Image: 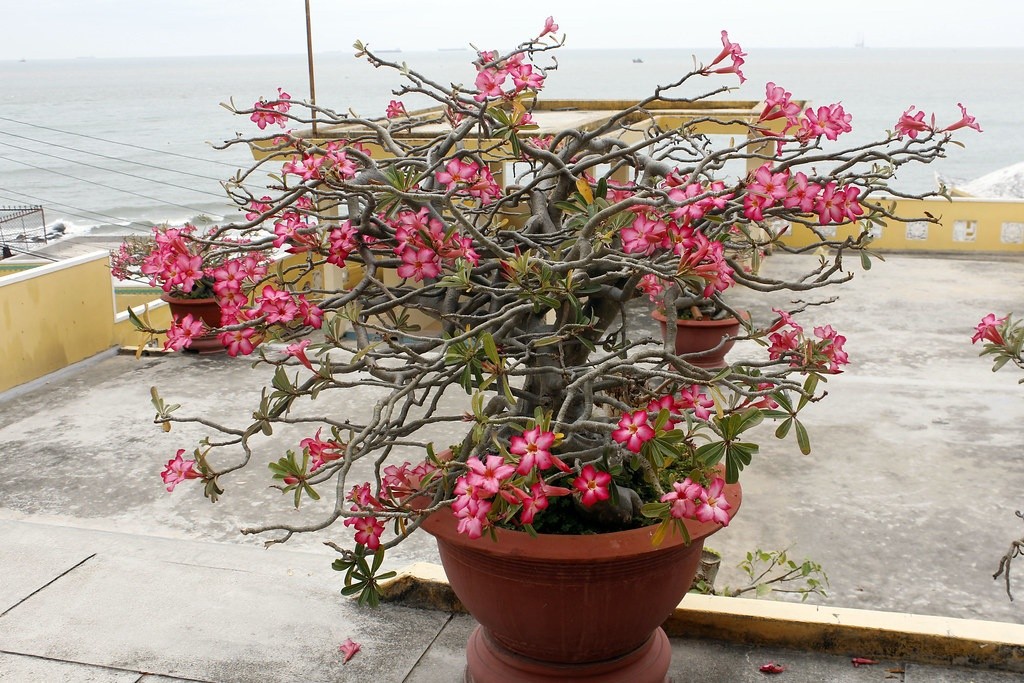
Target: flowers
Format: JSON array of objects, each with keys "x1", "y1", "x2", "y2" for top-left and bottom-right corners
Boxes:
[{"x1": 104, "y1": 11, "x2": 1024, "y2": 608}]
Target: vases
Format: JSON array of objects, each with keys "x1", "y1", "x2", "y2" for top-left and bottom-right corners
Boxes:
[{"x1": 399, "y1": 449, "x2": 742, "y2": 683}]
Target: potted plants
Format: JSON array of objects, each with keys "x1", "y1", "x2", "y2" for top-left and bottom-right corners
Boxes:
[
  {"x1": 650, "y1": 287, "x2": 749, "y2": 373},
  {"x1": 159, "y1": 276, "x2": 227, "y2": 356}
]
